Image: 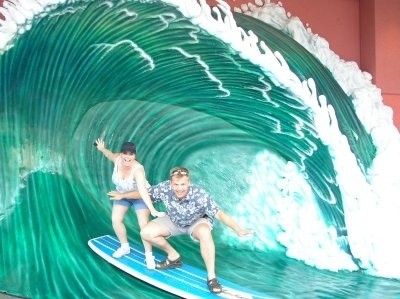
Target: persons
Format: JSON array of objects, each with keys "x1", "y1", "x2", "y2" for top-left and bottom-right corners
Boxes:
[
  {"x1": 96, "y1": 138, "x2": 166, "y2": 270},
  {"x1": 106, "y1": 168, "x2": 256, "y2": 293}
]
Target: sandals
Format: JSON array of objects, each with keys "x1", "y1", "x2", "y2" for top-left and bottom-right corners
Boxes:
[
  {"x1": 155, "y1": 256, "x2": 182, "y2": 269},
  {"x1": 207, "y1": 278, "x2": 222, "y2": 293}
]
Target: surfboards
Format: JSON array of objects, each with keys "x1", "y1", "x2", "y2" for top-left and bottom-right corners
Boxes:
[{"x1": 88, "y1": 234, "x2": 275, "y2": 299}]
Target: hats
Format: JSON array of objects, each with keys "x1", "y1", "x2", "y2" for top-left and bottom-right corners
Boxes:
[{"x1": 121, "y1": 142, "x2": 136, "y2": 153}]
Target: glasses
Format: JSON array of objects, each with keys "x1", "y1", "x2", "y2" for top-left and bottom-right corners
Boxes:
[{"x1": 171, "y1": 172, "x2": 188, "y2": 176}]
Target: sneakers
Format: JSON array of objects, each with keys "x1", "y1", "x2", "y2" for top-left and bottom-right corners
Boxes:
[
  {"x1": 146, "y1": 256, "x2": 155, "y2": 269},
  {"x1": 113, "y1": 246, "x2": 130, "y2": 258}
]
